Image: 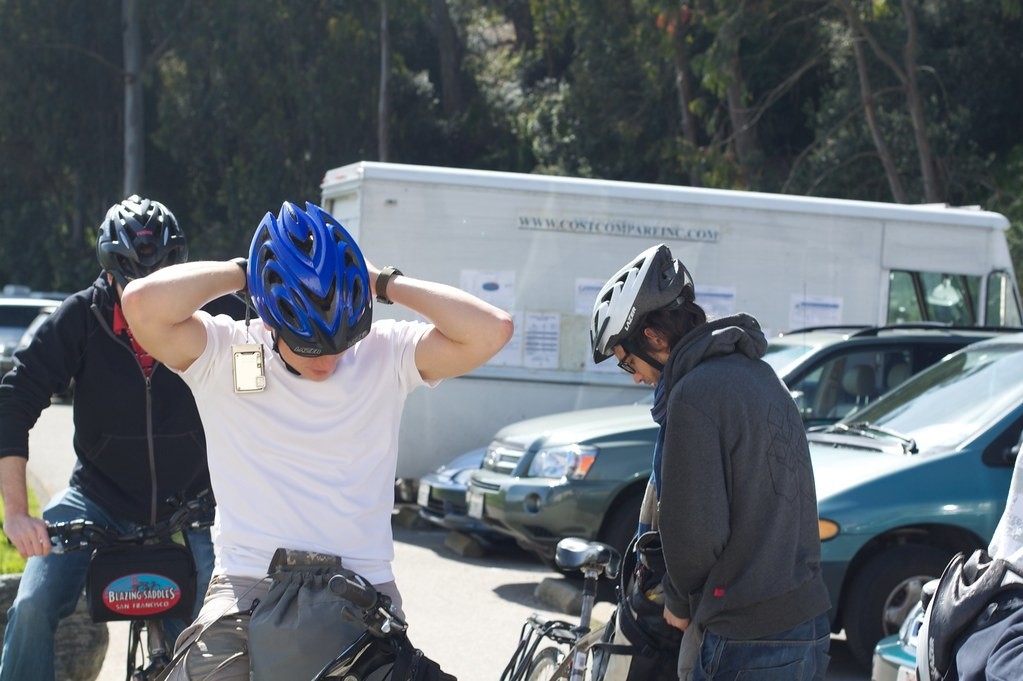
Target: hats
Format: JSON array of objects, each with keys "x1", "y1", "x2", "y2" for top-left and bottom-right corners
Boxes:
[{"x1": 588, "y1": 243, "x2": 695, "y2": 365}]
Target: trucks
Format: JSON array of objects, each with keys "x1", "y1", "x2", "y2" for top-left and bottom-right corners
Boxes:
[{"x1": 316, "y1": 158, "x2": 1022, "y2": 509}]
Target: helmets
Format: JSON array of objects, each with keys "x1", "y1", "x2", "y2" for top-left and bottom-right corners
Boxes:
[
  {"x1": 96, "y1": 193, "x2": 189, "y2": 291},
  {"x1": 245, "y1": 201, "x2": 373, "y2": 358}
]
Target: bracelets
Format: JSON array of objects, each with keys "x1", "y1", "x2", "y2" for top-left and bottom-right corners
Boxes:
[{"x1": 229, "y1": 255, "x2": 251, "y2": 294}]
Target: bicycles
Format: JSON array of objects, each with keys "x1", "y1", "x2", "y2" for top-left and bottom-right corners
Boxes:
[
  {"x1": 7, "y1": 486, "x2": 216, "y2": 681},
  {"x1": 500, "y1": 534, "x2": 623, "y2": 681}
]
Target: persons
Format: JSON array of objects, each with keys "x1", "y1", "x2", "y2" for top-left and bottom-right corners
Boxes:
[
  {"x1": 0, "y1": 191, "x2": 219, "y2": 681},
  {"x1": 590, "y1": 244, "x2": 838, "y2": 680},
  {"x1": 120, "y1": 201, "x2": 513, "y2": 681}
]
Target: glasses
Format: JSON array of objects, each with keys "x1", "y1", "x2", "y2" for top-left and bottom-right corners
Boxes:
[{"x1": 616, "y1": 351, "x2": 637, "y2": 376}]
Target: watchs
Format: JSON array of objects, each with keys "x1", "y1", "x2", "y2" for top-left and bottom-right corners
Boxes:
[{"x1": 374, "y1": 264, "x2": 403, "y2": 305}]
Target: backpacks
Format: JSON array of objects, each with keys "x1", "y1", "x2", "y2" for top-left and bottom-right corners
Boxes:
[{"x1": 153, "y1": 548, "x2": 379, "y2": 680}]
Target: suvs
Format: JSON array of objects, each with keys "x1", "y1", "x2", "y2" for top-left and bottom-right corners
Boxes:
[
  {"x1": 464, "y1": 317, "x2": 1023, "y2": 571},
  {"x1": 0, "y1": 298, "x2": 64, "y2": 379},
  {"x1": 810, "y1": 334, "x2": 1023, "y2": 673}
]
